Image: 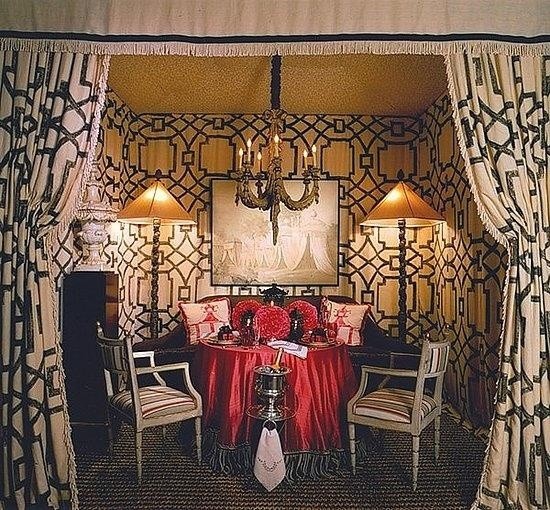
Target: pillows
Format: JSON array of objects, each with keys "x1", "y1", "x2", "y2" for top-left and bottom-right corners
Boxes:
[
  {"x1": 177, "y1": 296, "x2": 232, "y2": 346},
  {"x1": 317, "y1": 294, "x2": 372, "y2": 347}
]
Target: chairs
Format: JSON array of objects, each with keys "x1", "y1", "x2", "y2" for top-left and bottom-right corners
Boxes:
[
  {"x1": 341, "y1": 336, "x2": 452, "y2": 494},
  {"x1": 92, "y1": 320, "x2": 205, "y2": 484}
]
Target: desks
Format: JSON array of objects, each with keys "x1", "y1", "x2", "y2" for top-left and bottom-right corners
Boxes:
[{"x1": 241, "y1": 402, "x2": 296, "y2": 494}]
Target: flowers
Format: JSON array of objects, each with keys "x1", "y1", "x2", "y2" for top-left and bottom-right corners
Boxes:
[
  {"x1": 287, "y1": 300, "x2": 317, "y2": 332},
  {"x1": 228, "y1": 300, "x2": 263, "y2": 331},
  {"x1": 253, "y1": 305, "x2": 289, "y2": 341}
]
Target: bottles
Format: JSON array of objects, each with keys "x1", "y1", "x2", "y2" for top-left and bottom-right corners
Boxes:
[{"x1": 208, "y1": 308, "x2": 336, "y2": 348}]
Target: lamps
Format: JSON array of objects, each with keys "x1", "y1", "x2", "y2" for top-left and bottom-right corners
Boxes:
[
  {"x1": 113, "y1": 166, "x2": 199, "y2": 344},
  {"x1": 257, "y1": 282, "x2": 289, "y2": 308},
  {"x1": 358, "y1": 168, "x2": 446, "y2": 346},
  {"x1": 227, "y1": 53, "x2": 322, "y2": 245}
]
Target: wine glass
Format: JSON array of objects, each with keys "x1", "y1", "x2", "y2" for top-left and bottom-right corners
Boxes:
[{"x1": 252, "y1": 365, "x2": 291, "y2": 413}]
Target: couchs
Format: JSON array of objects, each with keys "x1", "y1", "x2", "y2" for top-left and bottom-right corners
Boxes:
[{"x1": 128, "y1": 291, "x2": 427, "y2": 403}]
